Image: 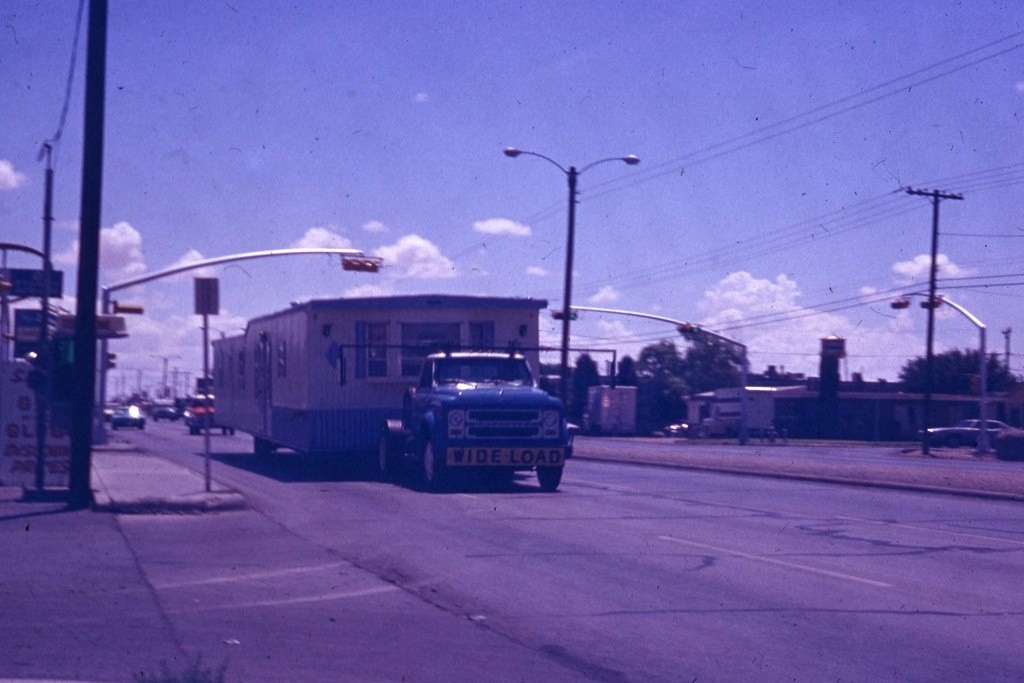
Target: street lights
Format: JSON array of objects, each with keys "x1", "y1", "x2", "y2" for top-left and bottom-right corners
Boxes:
[
  {"x1": 890, "y1": 291, "x2": 991, "y2": 459},
  {"x1": 502, "y1": 147, "x2": 642, "y2": 425},
  {"x1": 148, "y1": 353, "x2": 182, "y2": 398}
]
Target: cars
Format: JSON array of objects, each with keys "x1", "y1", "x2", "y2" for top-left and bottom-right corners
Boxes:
[
  {"x1": 664, "y1": 421, "x2": 709, "y2": 439},
  {"x1": 917, "y1": 418, "x2": 1014, "y2": 450},
  {"x1": 101, "y1": 392, "x2": 234, "y2": 435}
]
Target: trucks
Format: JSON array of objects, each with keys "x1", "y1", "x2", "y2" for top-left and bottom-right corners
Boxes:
[
  {"x1": 212, "y1": 293, "x2": 580, "y2": 492},
  {"x1": 581, "y1": 385, "x2": 638, "y2": 439}
]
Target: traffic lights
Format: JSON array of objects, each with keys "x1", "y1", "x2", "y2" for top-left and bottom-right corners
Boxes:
[
  {"x1": 105, "y1": 351, "x2": 117, "y2": 370},
  {"x1": 0, "y1": 278, "x2": 12, "y2": 294},
  {"x1": 342, "y1": 256, "x2": 380, "y2": 273},
  {"x1": 676, "y1": 324, "x2": 701, "y2": 336},
  {"x1": 550, "y1": 309, "x2": 577, "y2": 320}
]
